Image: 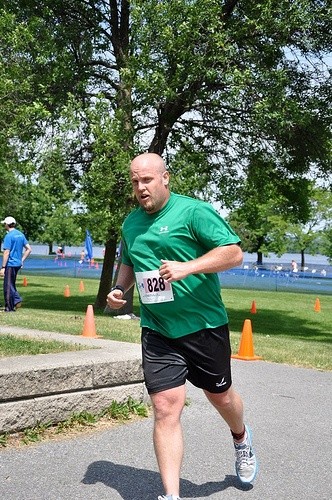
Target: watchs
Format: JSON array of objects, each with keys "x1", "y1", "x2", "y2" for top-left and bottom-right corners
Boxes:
[{"x1": 111, "y1": 285, "x2": 125, "y2": 295}]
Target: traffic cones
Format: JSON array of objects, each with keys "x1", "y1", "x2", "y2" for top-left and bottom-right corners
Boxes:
[
  {"x1": 23, "y1": 277, "x2": 27, "y2": 286},
  {"x1": 80, "y1": 304, "x2": 106, "y2": 339},
  {"x1": 231, "y1": 319, "x2": 262, "y2": 361},
  {"x1": 250, "y1": 299, "x2": 256, "y2": 314},
  {"x1": 79, "y1": 281, "x2": 84, "y2": 291},
  {"x1": 64, "y1": 285, "x2": 70, "y2": 297},
  {"x1": 55, "y1": 252, "x2": 119, "y2": 272},
  {"x1": 313, "y1": 297, "x2": 320, "y2": 312}
]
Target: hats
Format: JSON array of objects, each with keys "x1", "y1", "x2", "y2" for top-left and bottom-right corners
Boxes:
[{"x1": 1, "y1": 216, "x2": 16, "y2": 224}]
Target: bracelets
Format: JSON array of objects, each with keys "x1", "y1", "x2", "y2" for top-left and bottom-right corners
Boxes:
[{"x1": 2, "y1": 266, "x2": 5, "y2": 269}]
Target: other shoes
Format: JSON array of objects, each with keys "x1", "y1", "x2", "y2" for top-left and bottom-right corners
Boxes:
[{"x1": 16, "y1": 301, "x2": 22, "y2": 309}]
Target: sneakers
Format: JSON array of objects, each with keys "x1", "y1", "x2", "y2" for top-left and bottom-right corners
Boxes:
[
  {"x1": 234, "y1": 425, "x2": 256, "y2": 485},
  {"x1": 161, "y1": 494, "x2": 181, "y2": 500}
]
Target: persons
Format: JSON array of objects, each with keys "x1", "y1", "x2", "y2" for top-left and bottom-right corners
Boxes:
[
  {"x1": 106, "y1": 153, "x2": 259, "y2": 500},
  {"x1": 291, "y1": 259, "x2": 299, "y2": 281},
  {"x1": 0, "y1": 216, "x2": 32, "y2": 313},
  {"x1": 56, "y1": 243, "x2": 65, "y2": 259},
  {"x1": 78, "y1": 249, "x2": 86, "y2": 264}
]
